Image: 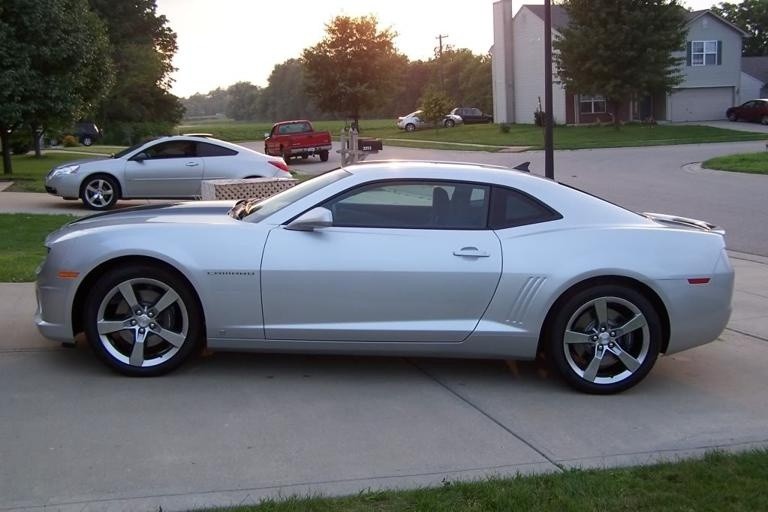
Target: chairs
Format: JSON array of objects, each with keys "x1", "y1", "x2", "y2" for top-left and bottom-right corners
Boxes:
[{"x1": 432, "y1": 187, "x2": 451, "y2": 223}]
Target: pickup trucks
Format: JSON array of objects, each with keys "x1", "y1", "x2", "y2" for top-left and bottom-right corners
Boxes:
[{"x1": 264, "y1": 119, "x2": 333, "y2": 165}]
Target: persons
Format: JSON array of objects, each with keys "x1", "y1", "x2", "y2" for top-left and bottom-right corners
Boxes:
[{"x1": 349, "y1": 123, "x2": 358, "y2": 136}]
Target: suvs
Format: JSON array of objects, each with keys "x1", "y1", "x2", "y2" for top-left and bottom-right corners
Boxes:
[
  {"x1": 450, "y1": 107, "x2": 494, "y2": 124},
  {"x1": 49, "y1": 121, "x2": 104, "y2": 147}
]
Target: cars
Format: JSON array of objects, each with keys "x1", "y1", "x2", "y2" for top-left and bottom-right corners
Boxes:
[
  {"x1": 43, "y1": 132, "x2": 293, "y2": 211},
  {"x1": 33, "y1": 159, "x2": 736, "y2": 394},
  {"x1": 396, "y1": 109, "x2": 464, "y2": 132},
  {"x1": 725, "y1": 97, "x2": 768, "y2": 127}
]
